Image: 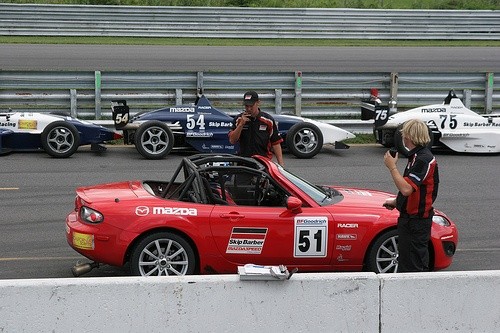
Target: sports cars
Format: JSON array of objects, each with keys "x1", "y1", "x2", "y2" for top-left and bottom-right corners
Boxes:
[{"x1": 66, "y1": 154, "x2": 458, "y2": 276}]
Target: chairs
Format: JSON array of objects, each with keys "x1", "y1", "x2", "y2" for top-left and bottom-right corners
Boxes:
[{"x1": 202, "y1": 172, "x2": 237, "y2": 205}]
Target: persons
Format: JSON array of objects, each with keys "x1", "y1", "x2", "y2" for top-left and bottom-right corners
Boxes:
[
  {"x1": 381, "y1": 119, "x2": 440, "y2": 273},
  {"x1": 227, "y1": 89, "x2": 285, "y2": 187}
]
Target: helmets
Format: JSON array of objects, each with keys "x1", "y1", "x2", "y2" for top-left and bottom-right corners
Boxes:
[{"x1": 204, "y1": 155, "x2": 232, "y2": 179}]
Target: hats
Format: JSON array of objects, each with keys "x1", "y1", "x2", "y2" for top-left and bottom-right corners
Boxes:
[{"x1": 242, "y1": 91, "x2": 259, "y2": 106}]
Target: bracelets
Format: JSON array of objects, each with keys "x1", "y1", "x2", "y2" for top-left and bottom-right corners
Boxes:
[{"x1": 390, "y1": 167, "x2": 397, "y2": 173}]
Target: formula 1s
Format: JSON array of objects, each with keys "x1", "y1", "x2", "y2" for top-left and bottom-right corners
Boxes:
[
  {"x1": 111, "y1": 95, "x2": 355, "y2": 158},
  {"x1": 360, "y1": 89, "x2": 498, "y2": 156},
  {"x1": 1, "y1": 108, "x2": 124, "y2": 159}
]
